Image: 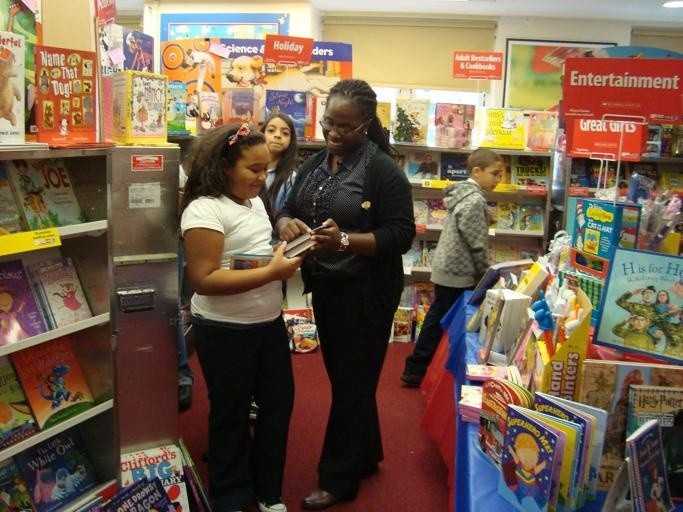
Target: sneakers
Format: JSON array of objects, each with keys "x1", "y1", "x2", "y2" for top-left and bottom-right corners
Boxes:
[
  {"x1": 179, "y1": 385, "x2": 192, "y2": 411},
  {"x1": 400, "y1": 368, "x2": 424, "y2": 385},
  {"x1": 257, "y1": 495, "x2": 287, "y2": 512}
]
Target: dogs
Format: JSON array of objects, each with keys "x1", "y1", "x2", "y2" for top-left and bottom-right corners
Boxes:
[{"x1": 225, "y1": 54, "x2": 332, "y2": 94}]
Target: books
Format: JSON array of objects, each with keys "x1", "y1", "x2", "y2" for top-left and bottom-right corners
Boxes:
[
  {"x1": 479, "y1": 108, "x2": 525, "y2": 150},
  {"x1": 459, "y1": 156, "x2": 683, "y2": 512},
  {"x1": 375, "y1": 100, "x2": 475, "y2": 343},
  {"x1": 526, "y1": 111, "x2": 559, "y2": 152},
  {"x1": 1, "y1": 32, "x2": 115, "y2": 151},
  {"x1": 1, "y1": 158, "x2": 209, "y2": 512}
]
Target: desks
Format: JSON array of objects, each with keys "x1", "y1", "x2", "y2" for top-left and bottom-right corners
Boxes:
[{"x1": 418, "y1": 290, "x2": 608, "y2": 512}]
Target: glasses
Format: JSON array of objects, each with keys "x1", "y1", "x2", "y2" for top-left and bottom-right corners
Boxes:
[{"x1": 318, "y1": 115, "x2": 371, "y2": 136}]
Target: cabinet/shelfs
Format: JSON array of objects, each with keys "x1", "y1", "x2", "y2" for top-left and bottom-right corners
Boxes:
[
  {"x1": 282, "y1": 136, "x2": 666, "y2": 315},
  {"x1": 0, "y1": 134, "x2": 115, "y2": 467}
]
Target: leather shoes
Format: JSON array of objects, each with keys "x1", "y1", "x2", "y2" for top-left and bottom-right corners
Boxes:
[{"x1": 304, "y1": 490, "x2": 335, "y2": 508}]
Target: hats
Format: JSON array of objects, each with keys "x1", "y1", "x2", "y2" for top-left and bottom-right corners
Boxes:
[
  {"x1": 187, "y1": 49, "x2": 192, "y2": 55},
  {"x1": 636, "y1": 309, "x2": 648, "y2": 317},
  {"x1": 645, "y1": 286, "x2": 656, "y2": 292}
]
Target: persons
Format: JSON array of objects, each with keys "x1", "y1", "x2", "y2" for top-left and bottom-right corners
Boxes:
[
  {"x1": 420, "y1": 289, "x2": 432, "y2": 304},
  {"x1": 401, "y1": 147, "x2": 504, "y2": 389},
  {"x1": 177, "y1": 79, "x2": 416, "y2": 511}
]
